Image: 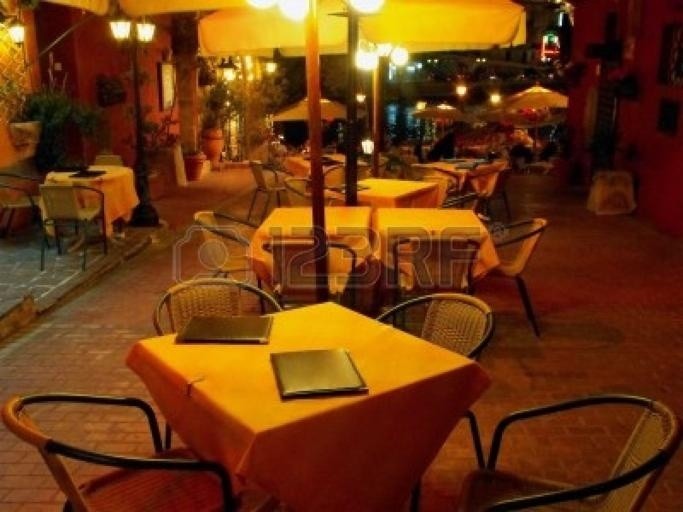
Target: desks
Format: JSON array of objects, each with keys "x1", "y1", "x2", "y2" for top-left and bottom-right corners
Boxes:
[{"x1": 44, "y1": 164, "x2": 132, "y2": 242}]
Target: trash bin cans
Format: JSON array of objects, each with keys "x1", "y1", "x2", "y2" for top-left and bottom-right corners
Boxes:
[{"x1": 586, "y1": 171, "x2": 633, "y2": 215}]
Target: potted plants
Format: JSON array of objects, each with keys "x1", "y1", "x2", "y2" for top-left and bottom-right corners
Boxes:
[{"x1": 199, "y1": 78, "x2": 244, "y2": 170}]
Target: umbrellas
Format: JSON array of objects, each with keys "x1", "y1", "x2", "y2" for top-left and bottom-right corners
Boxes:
[
  {"x1": 412, "y1": 100, "x2": 462, "y2": 120},
  {"x1": 264, "y1": 97, "x2": 367, "y2": 121},
  {"x1": 504, "y1": 80, "x2": 569, "y2": 109},
  {"x1": 47, "y1": 0, "x2": 449, "y2": 301},
  {"x1": 194, "y1": 0, "x2": 525, "y2": 206}
]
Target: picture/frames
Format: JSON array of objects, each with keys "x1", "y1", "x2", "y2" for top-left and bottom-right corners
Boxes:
[{"x1": 158, "y1": 62, "x2": 176, "y2": 112}]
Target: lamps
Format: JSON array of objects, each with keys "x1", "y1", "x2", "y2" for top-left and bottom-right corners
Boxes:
[{"x1": 9, "y1": 18, "x2": 25, "y2": 47}]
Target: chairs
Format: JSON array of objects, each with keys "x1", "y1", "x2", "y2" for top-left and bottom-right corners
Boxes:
[
  {"x1": 35, "y1": 184, "x2": 107, "y2": 271},
  {"x1": 152, "y1": 278, "x2": 273, "y2": 452},
  {"x1": 375, "y1": 293, "x2": 494, "y2": 476},
  {"x1": 461, "y1": 392, "x2": 682, "y2": 511},
  {"x1": 94, "y1": 155, "x2": 123, "y2": 166},
  {"x1": 190, "y1": 140, "x2": 549, "y2": 337},
  {"x1": 0, "y1": 173, "x2": 44, "y2": 245},
  {"x1": 0, "y1": 391, "x2": 240, "y2": 510}
]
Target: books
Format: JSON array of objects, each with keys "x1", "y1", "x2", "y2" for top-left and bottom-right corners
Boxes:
[
  {"x1": 269, "y1": 349, "x2": 366, "y2": 399},
  {"x1": 174, "y1": 315, "x2": 272, "y2": 345}
]
